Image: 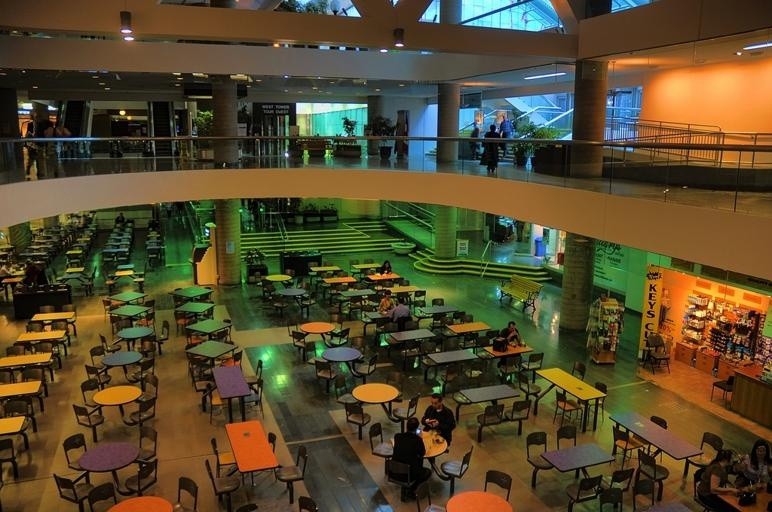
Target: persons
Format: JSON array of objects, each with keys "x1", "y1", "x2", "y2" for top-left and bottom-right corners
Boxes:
[
  {"x1": 380, "y1": 259, "x2": 393, "y2": 275},
  {"x1": 64, "y1": 212, "x2": 92, "y2": 230},
  {"x1": 113, "y1": 212, "x2": 125, "y2": 228},
  {"x1": 468, "y1": 113, "x2": 515, "y2": 174},
  {"x1": 512, "y1": 221, "x2": 526, "y2": 242},
  {"x1": 696, "y1": 439, "x2": 772, "y2": 512},
  {"x1": 377, "y1": 289, "x2": 411, "y2": 325},
  {"x1": 497, "y1": 321, "x2": 527, "y2": 369},
  {"x1": 22, "y1": 110, "x2": 77, "y2": 177},
  {"x1": 392, "y1": 393, "x2": 456, "y2": 499},
  {"x1": 0, "y1": 259, "x2": 41, "y2": 289}
]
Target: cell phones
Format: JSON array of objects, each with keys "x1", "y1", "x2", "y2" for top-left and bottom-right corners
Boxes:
[{"x1": 416, "y1": 428, "x2": 421, "y2": 435}]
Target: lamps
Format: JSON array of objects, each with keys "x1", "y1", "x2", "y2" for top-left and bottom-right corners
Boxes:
[
  {"x1": 393, "y1": 28, "x2": 405, "y2": 49},
  {"x1": 120, "y1": 0, "x2": 132, "y2": 34}
]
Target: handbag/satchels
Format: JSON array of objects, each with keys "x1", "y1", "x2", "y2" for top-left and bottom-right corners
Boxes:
[
  {"x1": 734, "y1": 469, "x2": 759, "y2": 488},
  {"x1": 738, "y1": 492, "x2": 758, "y2": 507}
]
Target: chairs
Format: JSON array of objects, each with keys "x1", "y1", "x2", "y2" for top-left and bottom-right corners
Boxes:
[
  {"x1": 135, "y1": 371, "x2": 160, "y2": 404},
  {"x1": 204, "y1": 383, "x2": 227, "y2": 424},
  {"x1": 438, "y1": 444, "x2": 476, "y2": 497},
  {"x1": 123, "y1": 457, "x2": 159, "y2": 498},
  {"x1": 682, "y1": 430, "x2": 724, "y2": 480},
  {"x1": 204, "y1": 457, "x2": 241, "y2": 510},
  {"x1": 523, "y1": 430, "x2": 552, "y2": 490},
  {"x1": 272, "y1": 219, "x2": 768, "y2": 466},
  {"x1": 172, "y1": 475, "x2": 200, "y2": 512},
  {"x1": 79, "y1": 378, "x2": 108, "y2": 418},
  {"x1": 1, "y1": 351, "x2": 78, "y2": 492},
  {"x1": 483, "y1": 468, "x2": 513, "y2": 502},
  {"x1": 51, "y1": 471, "x2": 95, "y2": 512},
  {"x1": 0, "y1": 218, "x2": 269, "y2": 387},
  {"x1": 414, "y1": 487, "x2": 448, "y2": 511},
  {"x1": 235, "y1": 502, "x2": 260, "y2": 512},
  {"x1": 297, "y1": 495, "x2": 321, "y2": 512},
  {"x1": 132, "y1": 424, "x2": 160, "y2": 478},
  {"x1": 596, "y1": 487, "x2": 625, "y2": 511},
  {"x1": 690, "y1": 466, "x2": 770, "y2": 512},
  {"x1": 274, "y1": 443, "x2": 310, "y2": 504},
  {"x1": 85, "y1": 480, "x2": 118, "y2": 511},
  {"x1": 634, "y1": 448, "x2": 673, "y2": 500},
  {"x1": 209, "y1": 436, "x2": 245, "y2": 486},
  {"x1": 129, "y1": 396, "x2": 159, "y2": 426},
  {"x1": 61, "y1": 431, "x2": 98, "y2": 489},
  {"x1": 628, "y1": 476, "x2": 656, "y2": 508},
  {"x1": 607, "y1": 466, "x2": 634, "y2": 494},
  {"x1": 241, "y1": 379, "x2": 265, "y2": 414},
  {"x1": 267, "y1": 430, "x2": 280, "y2": 454},
  {"x1": 384, "y1": 459, "x2": 419, "y2": 504},
  {"x1": 71, "y1": 402, "x2": 105, "y2": 443},
  {"x1": 563, "y1": 473, "x2": 604, "y2": 510}
]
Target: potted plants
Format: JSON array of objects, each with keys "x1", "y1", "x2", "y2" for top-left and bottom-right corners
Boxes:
[
  {"x1": 192, "y1": 110, "x2": 216, "y2": 163},
  {"x1": 281, "y1": 0, "x2": 329, "y2": 49},
  {"x1": 530, "y1": 127, "x2": 561, "y2": 166},
  {"x1": 510, "y1": 108, "x2": 537, "y2": 166},
  {"x1": 332, "y1": 116, "x2": 363, "y2": 157},
  {"x1": 287, "y1": 134, "x2": 303, "y2": 157},
  {"x1": 306, "y1": 135, "x2": 327, "y2": 156},
  {"x1": 370, "y1": 116, "x2": 399, "y2": 160}
]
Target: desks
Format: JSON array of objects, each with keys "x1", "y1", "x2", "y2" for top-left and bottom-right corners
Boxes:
[
  {"x1": 78, "y1": 441, "x2": 140, "y2": 490},
  {"x1": 224, "y1": 418, "x2": 280, "y2": 488},
  {"x1": 538, "y1": 444, "x2": 616, "y2": 474},
  {"x1": 624, "y1": 501, "x2": 695, "y2": 512},
  {"x1": 210, "y1": 364, "x2": 253, "y2": 423},
  {"x1": 102, "y1": 495, "x2": 175, "y2": 512},
  {"x1": 443, "y1": 490, "x2": 514, "y2": 512},
  {"x1": 92, "y1": 384, "x2": 145, "y2": 420}
]
